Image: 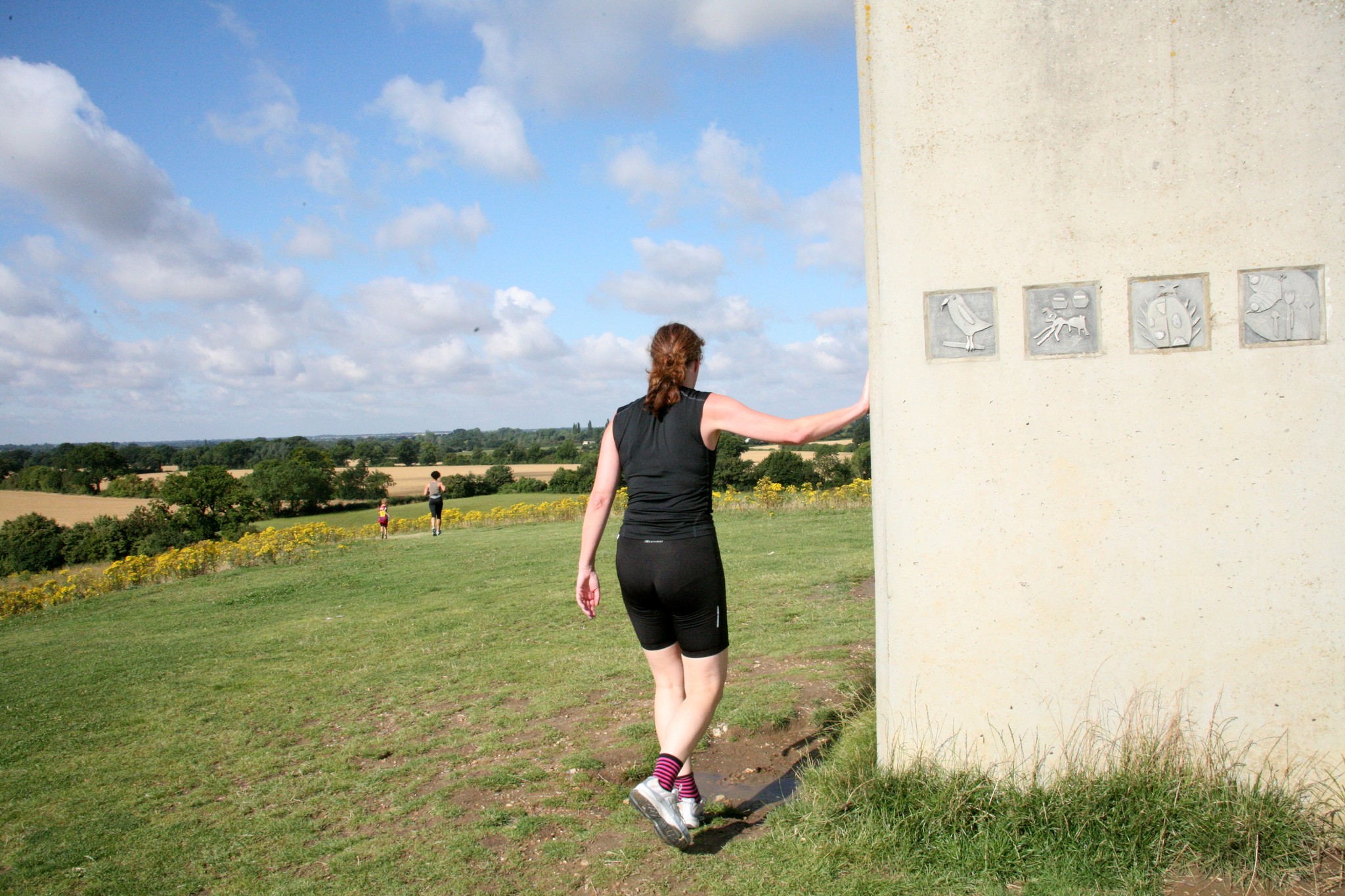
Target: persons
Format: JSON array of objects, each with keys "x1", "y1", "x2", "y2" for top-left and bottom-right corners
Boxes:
[
  {"x1": 378, "y1": 498, "x2": 391, "y2": 539},
  {"x1": 576, "y1": 323, "x2": 871, "y2": 850},
  {"x1": 423, "y1": 471, "x2": 446, "y2": 536}
]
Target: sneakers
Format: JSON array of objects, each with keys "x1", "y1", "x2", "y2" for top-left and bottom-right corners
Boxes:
[
  {"x1": 628, "y1": 776, "x2": 691, "y2": 850},
  {"x1": 676, "y1": 795, "x2": 707, "y2": 828}
]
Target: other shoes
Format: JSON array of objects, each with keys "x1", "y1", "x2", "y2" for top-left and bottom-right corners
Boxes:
[
  {"x1": 382, "y1": 532, "x2": 384, "y2": 539},
  {"x1": 385, "y1": 536, "x2": 386, "y2": 538},
  {"x1": 437, "y1": 530, "x2": 441, "y2": 534},
  {"x1": 432, "y1": 528, "x2": 436, "y2": 536}
]
